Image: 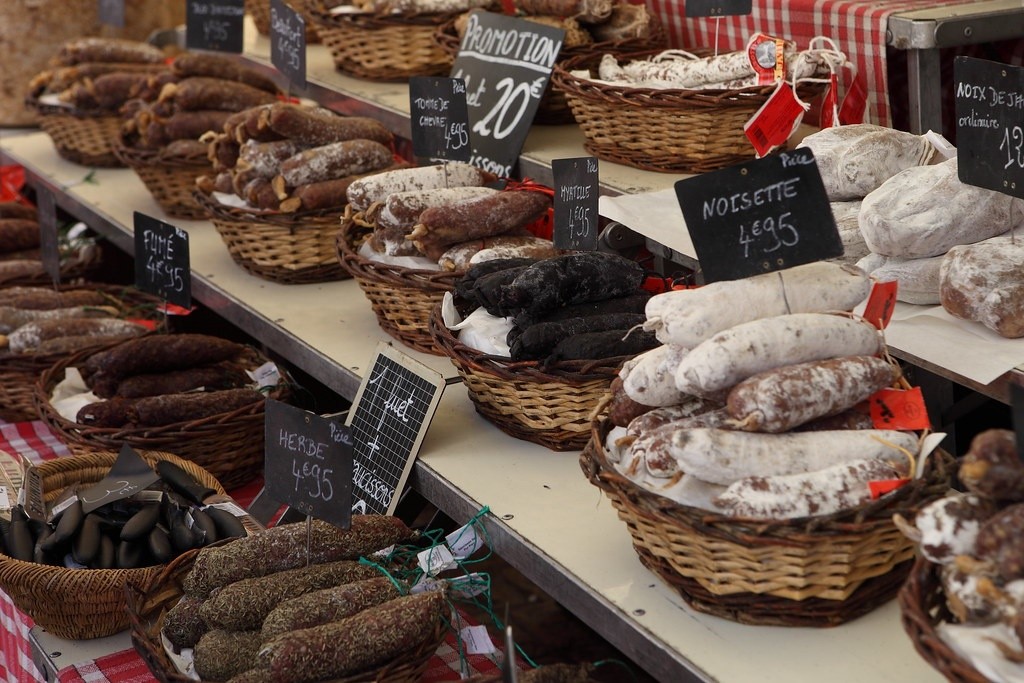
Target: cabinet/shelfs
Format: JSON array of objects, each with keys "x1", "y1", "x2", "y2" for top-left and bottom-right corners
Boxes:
[{"x1": 3, "y1": 1, "x2": 1023, "y2": 683}]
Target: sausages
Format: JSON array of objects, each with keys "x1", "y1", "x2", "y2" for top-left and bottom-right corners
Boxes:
[{"x1": 1, "y1": 0, "x2": 1024, "y2": 682}]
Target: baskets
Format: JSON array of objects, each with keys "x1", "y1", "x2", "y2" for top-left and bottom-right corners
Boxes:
[{"x1": 1, "y1": 0, "x2": 1024, "y2": 682}]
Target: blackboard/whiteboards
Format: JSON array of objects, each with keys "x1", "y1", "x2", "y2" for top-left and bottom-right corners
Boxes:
[
  {"x1": 407, "y1": 75, "x2": 471, "y2": 161},
  {"x1": 185, "y1": 0, "x2": 245, "y2": 54},
  {"x1": 675, "y1": 146, "x2": 845, "y2": 286},
  {"x1": 133, "y1": 211, "x2": 191, "y2": 310},
  {"x1": 270, "y1": 0, "x2": 307, "y2": 89},
  {"x1": 262, "y1": 395, "x2": 355, "y2": 532},
  {"x1": 342, "y1": 340, "x2": 445, "y2": 517},
  {"x1": 33, "y1": 179, "x2": 60, "y2": 285},
  {"x1": 444, "y1": 8, "x2": 566, "y2": 189},
  {"x1": 550, "y1": 155, "x2": 601, "y2": 251},
  {"x1": 98, "y1": 0, "x2": 126, "y2": 26},
  {"x1": 953, "y1": 54, "x2": 1024, "y2": 198}
]
